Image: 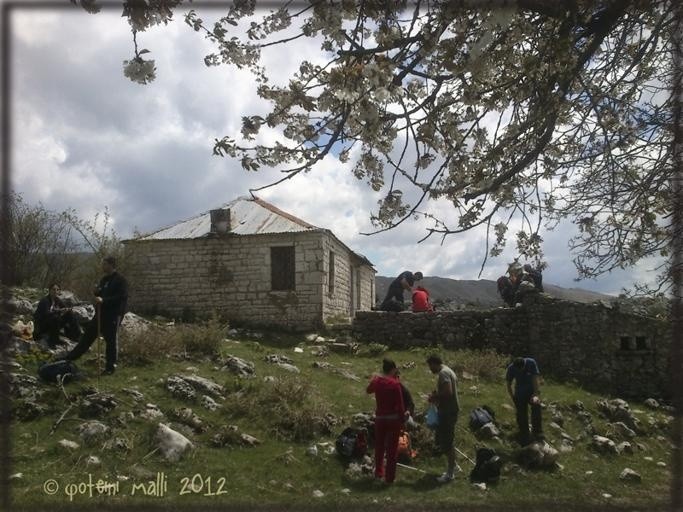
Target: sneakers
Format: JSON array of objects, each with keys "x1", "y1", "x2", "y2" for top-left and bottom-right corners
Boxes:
[
  {"x1": 436, "y1": 472, "x2": 455, "y2": 482},
  {"x1": 455, "y1": 464, "x2": 463, "y2": 473}
]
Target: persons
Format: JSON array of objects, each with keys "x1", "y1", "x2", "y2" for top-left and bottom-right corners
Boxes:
[
  {"x1": 424, "y1": 353, "x2": 464, "y2": 482},
  {"x1": 388, "y1": 367, "x2": 413, "y2": 421},
  {"x1": 382, "y1": 271, "x2": 424, "y2": 303},
  {"x1": 410, "y1": 288, "x2": 430, "y2": 312},
  {"x1": 35, "y1": 282, "x2": 80, "y2": 346},
  {"x1": 504, "y1": 355, "x2": 544, "y2": 447},
  {"x1": 523, "y1": 264, "x2": 542, "y2": 292},
  {"x1": 366, "y1": 360, "x2": 402, "y2": 484},
  {"x1": 62, "y1": 257, "x2": 129, "y2": 376}
]
